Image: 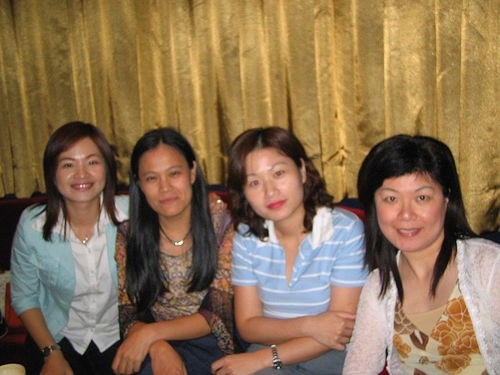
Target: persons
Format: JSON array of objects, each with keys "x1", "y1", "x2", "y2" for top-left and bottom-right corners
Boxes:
[
  {"x1": 209, "y1": 127, "x2": 375, "y2": 375},
  {"x1": 342, "y1": 132, "x2": 500, "y2": 375},
  {"x1": 10, "y1": 120, "x2": 135, "y2": 375},
  {"x1": 110, "y1": 127, "x2": 238, "y2": 375}
]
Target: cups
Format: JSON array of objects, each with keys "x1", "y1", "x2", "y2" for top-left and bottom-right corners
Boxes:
[{"x1": 0, "y1": 364, "x2": 26, "y2": 375}]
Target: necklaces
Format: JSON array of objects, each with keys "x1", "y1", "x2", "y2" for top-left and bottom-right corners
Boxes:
[
  {"x1": 68, "y1": 217, "x2": 99, "y2": 247},
  {"x1": 156, "y1": 221, "x2": 193, "y2": 246}
]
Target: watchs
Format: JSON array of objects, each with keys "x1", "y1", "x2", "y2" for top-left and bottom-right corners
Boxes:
[
  {"x1": 271, "y1": 344, "x2": 283, "y2": 370},
  {"x1": 41, "y1": 344, "x2": 61, "y2": 358}
]
